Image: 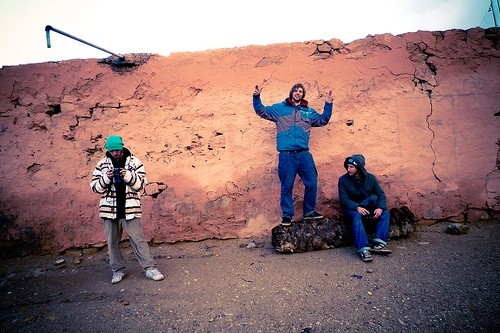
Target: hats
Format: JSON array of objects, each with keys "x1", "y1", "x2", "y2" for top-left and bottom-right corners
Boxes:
[
  {"x1": 347, "y1": 158, "x2": 358, "y2": 171},
  {"x1": 105, "y1": 135, "x2": 124, "y2": 152}
]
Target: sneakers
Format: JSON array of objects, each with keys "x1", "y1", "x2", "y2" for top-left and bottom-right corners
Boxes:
[
  {"x1": 304, "y1": 211, "x2": 324, "y2": 220},
  {"x1": 282, "y1": 218, "x2": 291, "y2": 226},
  {"x1": 111, "y1": 269, "x2": 127, "y2": 283},
  {"x1": 146, "y1": 266, "x2": 164, "y2": 281},
  {"x1": 372, "y1": 241, "x2": 392, "y2": 254},
  {"x1": 360, "y1": 249, "x2": 374, "y2": 261}
]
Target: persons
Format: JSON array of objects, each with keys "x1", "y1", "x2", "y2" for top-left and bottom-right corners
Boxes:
[
  {"x1": 253, "y1": 83, "x2": 335, "y2": 226},
  {"x1": 90, "y1": 135, "x2": 164, "y2": 285},
  {"x1": 338, "y1": 154, "x2": 393, "y2": 261}
]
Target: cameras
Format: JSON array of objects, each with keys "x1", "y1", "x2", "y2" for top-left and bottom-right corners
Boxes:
[{"x1": 109, "y1": 167, "x2": 121, "y2": 175}]
